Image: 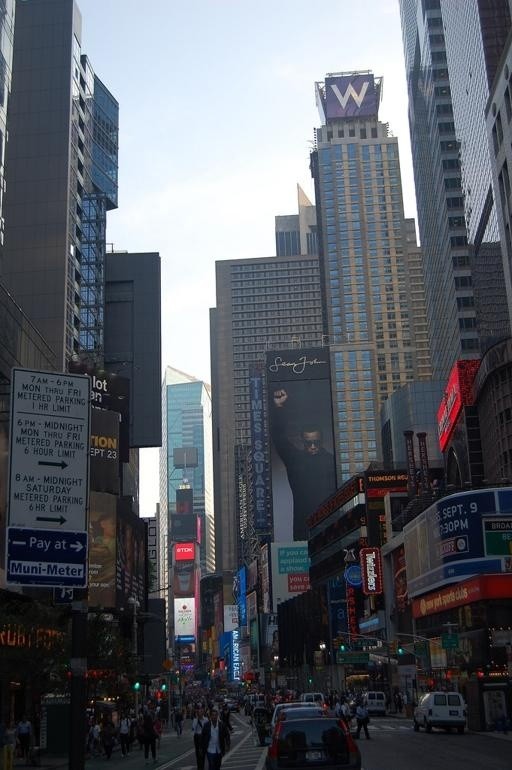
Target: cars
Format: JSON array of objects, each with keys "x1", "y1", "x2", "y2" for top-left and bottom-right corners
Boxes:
[
  {"x1": 215, "y1": 689, "x2": 240, "y2": 712},
  {"x1": 267, "y1": 693, "x2": 361, "y2": 770}
]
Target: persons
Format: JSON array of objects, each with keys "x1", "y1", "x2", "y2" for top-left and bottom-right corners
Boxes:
[
  {"x1": 2, "y1": 714, "x2": 32, "y2": 760},
  {"x1": 272, "y1": 389, "x2": 337, "y2": 541},
  {"x1": 83, "y1": 691, "x2": 233, "y2": 769},
  {"x1": 91, "y1": 513, "x2": 146, "y2": 579},
  {"x1": 325, "y1": 688, "x2": 408, "y2": 740}
]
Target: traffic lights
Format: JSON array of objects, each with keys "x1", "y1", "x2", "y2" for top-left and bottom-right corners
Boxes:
[
  {"x1": 336, "y1": 636, "x2": 347, "y2": 653},
  {"x1": 396, "y1": 640, "x2": 404, "y2": 655},
  {"x1": 133, "y1": 681, "x2": 140, "y2": 691},
  {"x1": 161, "y1": 684, "x2": 165, "y2": 690}
]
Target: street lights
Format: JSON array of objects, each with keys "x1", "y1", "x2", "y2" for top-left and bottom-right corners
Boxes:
[{"x1": 128, "y1": 592, "x2": 141, "y2": 720}]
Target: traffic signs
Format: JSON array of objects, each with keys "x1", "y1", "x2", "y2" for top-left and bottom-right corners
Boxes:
[{"x1": 4, "y1": 367, "x2": 93, "y2": 589}]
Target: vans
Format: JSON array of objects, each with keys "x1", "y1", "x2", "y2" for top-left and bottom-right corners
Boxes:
[
  {"x1": 413, "y1": 692, "x2": 468, "y2": 733},
  {"x1": 362, "y1": 692, "x2": 387, "y2": 716},
  {"x1": 247, "y1": 694, "x2": 265, "y2": 712}
]
